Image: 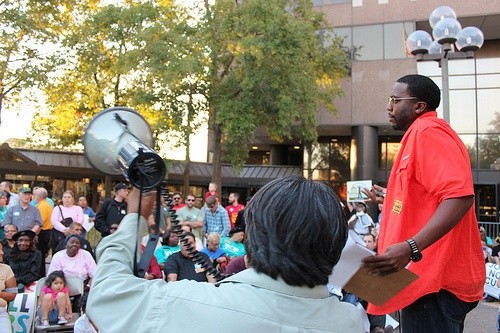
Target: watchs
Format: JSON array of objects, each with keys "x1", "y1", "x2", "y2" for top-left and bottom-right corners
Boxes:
[{"x1": 406, "y1": 237, "x2": 423, "y2": 262}]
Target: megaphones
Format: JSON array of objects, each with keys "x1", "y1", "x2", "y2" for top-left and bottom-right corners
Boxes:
[{"x1": 83, "y1": 107, "x2": 166, "y2": 191}]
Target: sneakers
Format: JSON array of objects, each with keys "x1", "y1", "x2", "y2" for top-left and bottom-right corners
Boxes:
[
  {"x1": 41, "y1": 319, "x2": 50, "y2": 329},
  {"x1": 58, "y1": 317, "x2": 67, "y2": 324}
]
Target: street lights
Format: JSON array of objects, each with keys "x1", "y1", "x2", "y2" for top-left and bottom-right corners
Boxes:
[{"x1": 406, "y1": 6, "x2": 484, "y2": 124}]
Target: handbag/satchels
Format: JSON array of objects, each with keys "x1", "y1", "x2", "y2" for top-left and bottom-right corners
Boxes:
[{"x1": 52, "y1": 205, "x2": 74, "y2": 242}]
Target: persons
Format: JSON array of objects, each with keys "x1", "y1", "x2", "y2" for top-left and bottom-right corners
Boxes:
[
  {"x1": 331, "y1": 173, "x2": 344, "y2": 197},
  {"x1": 358, "y1": 233, "x2": 394, "y2": 333},
  {"x1": 199, "y1": 233, "x2": 231, "y2": 270},
  {"x1": 139, "y1": 244, "x2": 163, "y2": 280},
  {"x1": 141, "y1": 182, "x2": 254, "y2": 258},
  {"x1": 0, "y1": 239, "x2": 19, "y2": 333},
  {"x1": 8, "y1": 230, "x2": 43, "y2": 293},
  {"x1": 44, "y1": 234, "x2": 98, "y2": 312},
  {"x1": 95, "y1": 182, "x2": 131, "y2": 238},
  {"x1": 477, "y1": 225, "x2": 500, "y2": 302},
  {"x1": 154, "y1": 229, "x2": 181, "y2": 271},
  {"x1": 163, "y1": 232, "x2": 217, "y2": 283},
  {"x1": 331, "y1": 202, "x2": 383, "y2": 305},
  {"x1": 36, "y1": 270, "x2": 72, "y2": 326},
  {"x1": 0, "y1": 181, "x2": 96, "y2": 260},
  {"x1": 223, "y1": 253, "x2": 250, "y2": 275},
  {"x1": 360, "y1": 74, "x2": 487, "y2": 333},
  {"x1": 74, "y1": 292, "x2": 99, "y2": 333},
  {"x1": 85, "y1": 175, "x2": 366, "y2": 333}
]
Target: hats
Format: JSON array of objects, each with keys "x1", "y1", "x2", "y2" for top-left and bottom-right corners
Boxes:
[
  {"x1": 115, "y1": 182, "x2": 132, "y2": 189},
  {"x1": 12, "y1": 229, "x2": 36, "y2": 241},
  {"x1": 64, "y1": 234, "x2": 82, "y2": 245},
  {"x1": 19, "y1": 187, "x2": 31, "y2": 192},
  {"x1": 33, "y1": 187, "x2": 48, "y2": 197}
]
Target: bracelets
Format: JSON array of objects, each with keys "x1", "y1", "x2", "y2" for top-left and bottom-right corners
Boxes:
[{"x1": 87, "y1": 284, "x2": 91, "y2": 288}]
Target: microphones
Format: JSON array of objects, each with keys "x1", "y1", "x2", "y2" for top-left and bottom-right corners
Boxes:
[{"x1": 371, "y1": 190, "x2": 385, "y2": 197}]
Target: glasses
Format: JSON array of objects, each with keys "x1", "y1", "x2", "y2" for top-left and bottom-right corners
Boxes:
[
  {"x1": 207, "y1": 206, "x2": 214, "y2": 208},
  {"x1": 174, "y1": 198, "x2": 180, "y2": 200},
  {"x1": 388, "y1": 96, "x2": 418, "y2": 103},
  {"x1": 187, "y1": 199, "x2": 194, "y2": 202}
]
